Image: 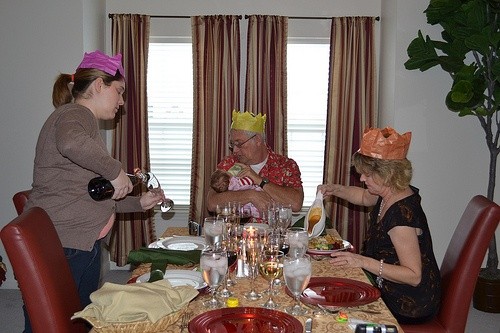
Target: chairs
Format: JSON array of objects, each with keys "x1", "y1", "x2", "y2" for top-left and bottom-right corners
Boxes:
[
  {"x1": 400, "y1": 195, "x2": 500, "y2": 333},
  {"x1": 0, "y1": 190, "x2": 86, "y2": 333}
]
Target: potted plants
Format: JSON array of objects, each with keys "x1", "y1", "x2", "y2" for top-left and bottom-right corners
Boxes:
[{"x1": 404, "y1": 0, "x2": 500, "y2": 315}]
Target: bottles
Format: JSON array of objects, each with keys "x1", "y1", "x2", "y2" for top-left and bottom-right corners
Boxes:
[
  {"x1": 227, "y1": 298, "x2": 238, "y2": 308},
  {"x1": 88, "y1": 173, "x2": 143, "y2": 201}
]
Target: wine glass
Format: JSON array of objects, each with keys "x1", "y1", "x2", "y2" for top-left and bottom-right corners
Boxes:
[
  {"x1": 200, "y1": 201, "x2": 312, "y2": 317},
  {"x1": 143, "y1": 171, "x2": 174, "y2": 213}
]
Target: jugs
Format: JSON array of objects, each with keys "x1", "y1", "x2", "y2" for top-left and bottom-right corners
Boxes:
[{"x1": 304, "y1": 190, "x2": 326, "y2": 241}]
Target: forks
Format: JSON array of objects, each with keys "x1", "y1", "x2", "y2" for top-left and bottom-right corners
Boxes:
[
  {"x1": 179, "y1": 313, "x2": 190, "y2": 333},
  {"x1": 319, "y1": 304, "x2": 381, "y2": 315}
]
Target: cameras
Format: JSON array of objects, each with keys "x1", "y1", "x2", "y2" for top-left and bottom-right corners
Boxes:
[{"x1": 189, "y1": 221, "x2": 201, "y2": 236}]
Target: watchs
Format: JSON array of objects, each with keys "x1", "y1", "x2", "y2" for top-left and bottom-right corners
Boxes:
[{"x1": 260, "y1": 178, "x2": 270, "y2": 188}]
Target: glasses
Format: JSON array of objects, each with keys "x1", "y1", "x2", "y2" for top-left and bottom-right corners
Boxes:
[{"x1": 227, "y1": 134, "x2": 257, "y2": 148}]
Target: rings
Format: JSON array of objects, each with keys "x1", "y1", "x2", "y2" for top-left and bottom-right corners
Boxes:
[{"x1": 346, "y1": 260, "x2": 348, "y2": 262}]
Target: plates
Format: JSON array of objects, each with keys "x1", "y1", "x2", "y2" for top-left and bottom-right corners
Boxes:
[
  {"x1": 147, "y1": 235, "x2": 207, "y2": 255},
  {"x1": 306, "y1": 237, "x2": 352, "y2": 254},
  {"x1": 189, "y1": 307, "x2": 303, "y2": 333},
  {"x1": 285, "y1": 277, "x2": 380, "y2": 307},
  {"x1": 126, "y1": 269, "x2": 209, "y2": 290}
]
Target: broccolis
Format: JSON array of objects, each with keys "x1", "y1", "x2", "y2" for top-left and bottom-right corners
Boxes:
[{"x1": 316, "y1": 243, "x2": 333, "y2": 250}]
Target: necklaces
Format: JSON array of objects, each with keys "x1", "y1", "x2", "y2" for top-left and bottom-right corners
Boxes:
[
  {"x1": 378, "y1": 197, "x2": 387, "y2": 216},
  {"x1": 377, "y1": 260, "x2": 383, "y2": 288}
]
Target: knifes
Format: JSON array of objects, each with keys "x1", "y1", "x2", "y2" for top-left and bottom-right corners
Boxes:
[{"x1": 305, "y1": 318, "x2": 313, "y2": 333}]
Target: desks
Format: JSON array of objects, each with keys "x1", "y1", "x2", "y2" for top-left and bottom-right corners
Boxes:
[{"x1": 90, "y1": 227, "x2": 403, "y2": 333}]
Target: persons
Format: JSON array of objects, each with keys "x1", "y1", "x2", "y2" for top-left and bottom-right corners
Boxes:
[
  {"x1": 210, "y1": 163, "x2": 279, "y2": 219},
  {"x1": 207, "y1": 109, "x2": 304, "y2": 226},
  {"x1": 21, "y1": 50, "x2": 166, "y2": 333},
  {"x1": 316, "y1": 127, "x2": 442, "y2": 325}
]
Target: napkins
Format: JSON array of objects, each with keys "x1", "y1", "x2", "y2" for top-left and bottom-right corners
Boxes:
[{"x1": 71, "y1": 278, "x2": 199, "y2": 328}]
